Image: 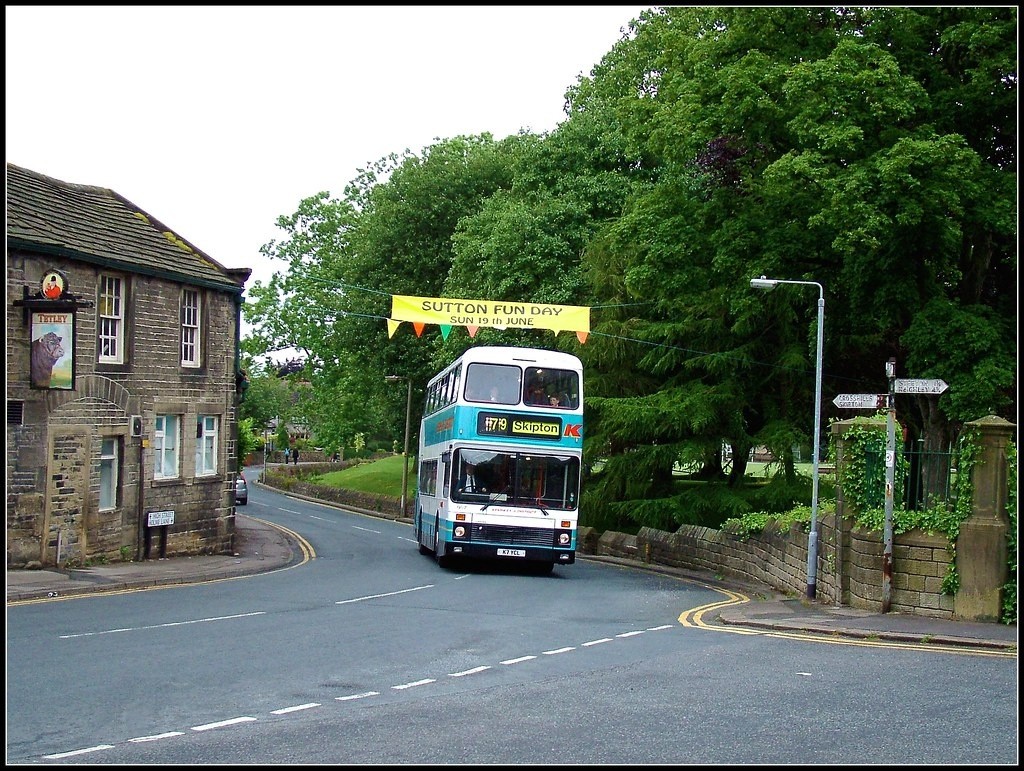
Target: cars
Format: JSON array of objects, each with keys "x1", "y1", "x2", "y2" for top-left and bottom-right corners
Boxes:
[{"x1": 235, "y1": 474, "x2": 249, "y2": 505}]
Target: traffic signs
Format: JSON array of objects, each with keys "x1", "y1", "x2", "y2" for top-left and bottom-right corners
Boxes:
[
  {"x1": 835, "y1": 393, "x2": 878, "y2": 408},
  {"x1": 894, "y1": 378, "x2": 950, "y2": 395}
]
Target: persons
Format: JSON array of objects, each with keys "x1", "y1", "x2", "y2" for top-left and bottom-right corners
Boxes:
[
  {"x1": 454, "y1": 463, "x2": 488, "y2": 501},
  {"x1": 468, "y1": 372, "x2": 571, "y2": 407},
  {"x1": 284, "y1": 446, "x2": 300, "y2": 466}
]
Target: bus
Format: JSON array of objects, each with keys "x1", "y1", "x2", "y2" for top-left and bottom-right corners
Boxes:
[{"x1": 414, "y1": 347, "x2": 584, "y2": 574}]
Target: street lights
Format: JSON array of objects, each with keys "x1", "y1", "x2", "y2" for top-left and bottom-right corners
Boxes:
[
  {"x1": 383, "y1": 376, "x2": 412, "y2": 518},
  {"x1": 750, "y1": 278, "x2": 819, "y2": 599},
  {"x1": 263, "y1": 418, "x2": 277, "y2": 484}
]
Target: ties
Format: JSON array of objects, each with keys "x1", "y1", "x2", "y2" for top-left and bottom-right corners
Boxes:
[{"x1": 470, "y1": 476, "x2": 473, "y2": 492}]
